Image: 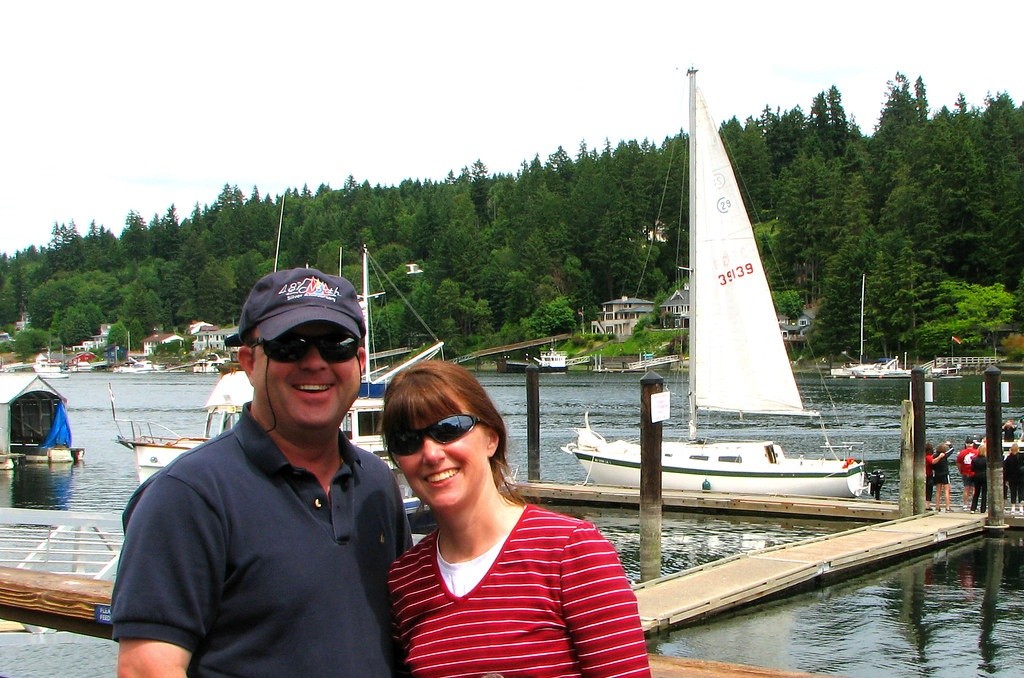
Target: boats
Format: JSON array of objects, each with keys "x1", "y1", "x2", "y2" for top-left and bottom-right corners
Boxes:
[
  {"x1": 192, "y1": 352, "x2": 229, "y2": 373},
  {"x1": 33, "y1": 364, "x2": 61, "y2": 374},
  {"x1": 113, "y1": 360, "x2": 154, "y2": 374},
  {"x1": 0, "y1": 370, "x2": 86, "y2": 471},
  {"x1": 68, "y1": 361, "x2": 92, "y2": 373},
  {"x1": 505, "y1": 347, "x2": 569, "y2": 375}
]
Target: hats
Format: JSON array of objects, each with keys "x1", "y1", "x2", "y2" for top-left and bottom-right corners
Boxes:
[
  {"x1": 965, "y1": 438, "x2": 973, "y2": 444},
  {"x1": 224, "y1": 268, "x2": 366, "y2": 348}
]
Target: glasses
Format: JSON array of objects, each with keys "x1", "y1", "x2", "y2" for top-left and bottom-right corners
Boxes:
[
  {"x1": 389, "y1": 415, "x2": 490, "y2": 456},
  {"x1": 248, "y1": 332, "x2": 358, "y2": 363}
]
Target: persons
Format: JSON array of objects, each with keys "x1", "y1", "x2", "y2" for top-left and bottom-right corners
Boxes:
[
  {"x1": 1002, "y1": 419, "x2": 1024, "y2": 442},
  {"x1": 926, "y1": 441, "x2": 955, "y2": 513},
  {"x1": 110, "y1": 268, "x2": 413, "y2": 678},
  {"x1": 956, "y1": 436, "x2": 987, "y2": 513},
  {"x1": 1002, "y1": 444, "x2": 1024, "y2": 515},
  {"x1": 382, "y1": 360, "x2": 677, "y2": 678}
]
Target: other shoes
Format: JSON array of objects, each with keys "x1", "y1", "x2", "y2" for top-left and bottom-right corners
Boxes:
[
  {"x1": 971, "y1": 510, "x2": 974, "y2": 514},
  {"x1": 926, "y1": 508, "x2": 932, "y2": 510},
  {"x1": 963, "y1": 505, "x2": 970, "y2": 510},
  {"x1": 945, "y1": 508, "x2": 953, "y2": 512},
  {"x1": 936, "y1": 508, "x2": 941, "y2": 511}
]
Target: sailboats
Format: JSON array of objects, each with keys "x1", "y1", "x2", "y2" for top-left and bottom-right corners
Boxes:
[
  {"x1": 106, "y1": 188, "x2": 448, "y2": 536},
  {"x1": 558, "y1": 58, "x2": 887, "y2": 503},
  {"x1": 828, "y1": 272, "x2": 912, "y2": 378}
]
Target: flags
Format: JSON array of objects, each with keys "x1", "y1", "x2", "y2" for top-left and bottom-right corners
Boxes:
[{"x1": 952, "y1": 335, "x2": 962, "y2": 344}]
